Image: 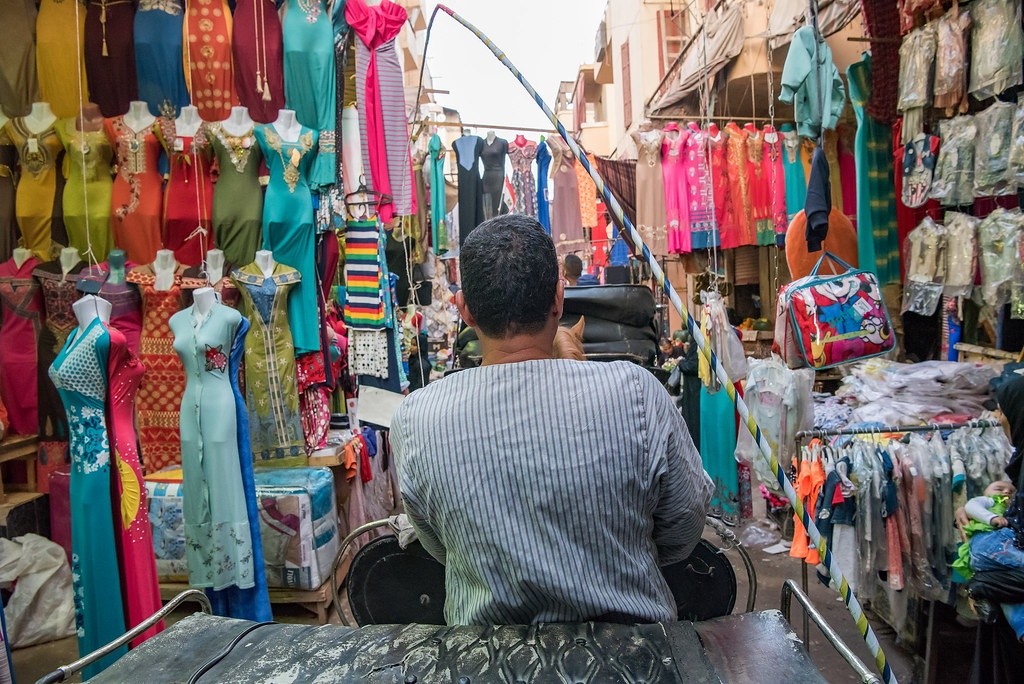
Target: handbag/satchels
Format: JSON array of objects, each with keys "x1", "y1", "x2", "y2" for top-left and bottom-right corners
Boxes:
[
  {"x1": 771, "y1": 253, "x2": 897, "y2": 371},
  {"x1": 409, "y1": 265, "x2": 433, "y2": 305}
]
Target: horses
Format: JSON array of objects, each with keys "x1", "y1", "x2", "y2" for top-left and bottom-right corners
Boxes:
[{"x1": 552, "y1": 315, "x2": 585, "y2": 363}]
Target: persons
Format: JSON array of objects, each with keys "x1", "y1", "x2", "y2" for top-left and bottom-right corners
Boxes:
[
  {"x1": 695, "y1": 322, "x2": 753, "y2": 528},
  {"x1": 657, "y1": 336, "x2": 700, "y2": 449},
  {"x1": 408, "y1": 333, "x2": 431, "y2": 392},
  {"x1": 429, "y1": 132, "x2": 450, "y2": 254},
  {"x1": 846, "y1": 49, "x2": 901, "y2": 287},
  {"x1": 0, "y1": 247, "x2": 309, "y2": 472},
  {"x1": 396, "y1": 303, "x2": 423, "y2": 376},
  {"x1": 450, "y1": 128, "x2": 585, "y2": 254},
  {"x1": 629, "y1": 120, "x2": 855, "y2": 257},
  {"x1": 167, "y1": 287, "x2": 274, "y2": 623},
  {"x1": 49, "y1": 295, "x2": 165, "y2": 682},
  {"x1": 402, "y1": 138, "x2": 428, "y2": 265},
  {"x1": 344, "y1": 0, "x2": 420, "y2": 218},
  {"x1": 0, "y1": 102, "x2": 320, "y2": 357},
  {"x1": 561, "y1": 252, "x2": 586, "y2": 287},
  {"x1": 386, "y1": 226, "x2": 415, "y2": 306},
  {"x1": 590, "y1": 199, "x2": 609, "y2": 269},
  {"x1": 0, "y1": 0, "x2": 337, "y2": 190},
  {"x1": 951, "y1": 362, "x2": 1024, "y2": 684},
  {"x1": 387, "y1": 213, "x2": 715, "y2": 629}
]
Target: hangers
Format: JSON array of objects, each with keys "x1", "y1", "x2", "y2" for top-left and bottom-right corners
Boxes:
[
  {"x1": 897, "y1": 0, "x2": 1024, "y2": 245},
  {"x1": 343, "y1": 173, "x2": 394, "y2": 206},
  {"x1": 793, "y1": 417, "x2": 1013, "y2": 487}
]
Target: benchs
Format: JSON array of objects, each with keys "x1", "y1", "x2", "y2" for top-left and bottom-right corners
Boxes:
[{"x1": 32, "y1": 578, "x2": 887, "y2": 684}]
